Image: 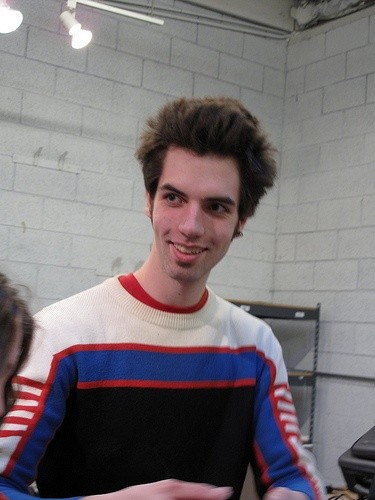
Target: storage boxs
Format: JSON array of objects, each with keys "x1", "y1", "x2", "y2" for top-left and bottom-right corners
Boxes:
[{"x1": 339, "y1": 435, "x2": 375, "y2": 499}]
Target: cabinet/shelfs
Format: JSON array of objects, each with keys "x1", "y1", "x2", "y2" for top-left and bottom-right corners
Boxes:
[{"x1": 221, "y1": 298, "x2": 321, "y2": 454}]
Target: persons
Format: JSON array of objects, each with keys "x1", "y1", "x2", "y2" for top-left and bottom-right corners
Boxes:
[{"x1": 0, "y1": 97, "x2": 326, "y2": 500}]
